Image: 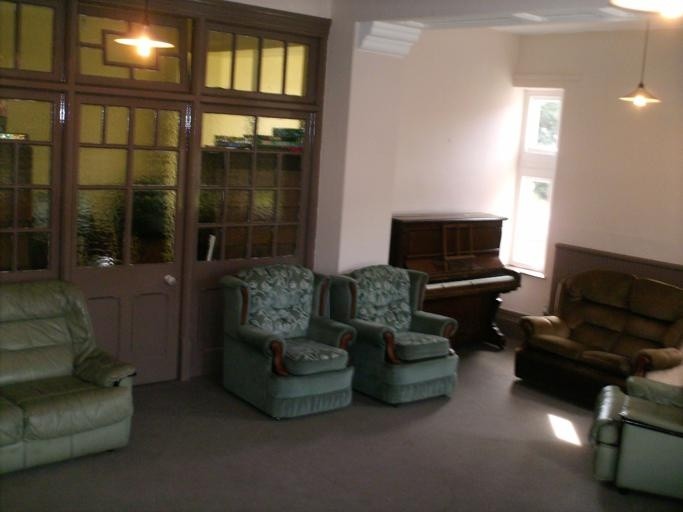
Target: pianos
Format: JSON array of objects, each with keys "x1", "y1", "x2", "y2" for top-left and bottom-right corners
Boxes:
[{"x1": 389, "y1": 211, "x2": 522, "y2": 350}]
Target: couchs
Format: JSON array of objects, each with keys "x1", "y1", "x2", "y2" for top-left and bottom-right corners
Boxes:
[
  {"x1": 513, "y1": 268, "x2": 682, "y2": 413},
  {"x1": 0, "y1": 276, "x2": 138, "y2": 473}
]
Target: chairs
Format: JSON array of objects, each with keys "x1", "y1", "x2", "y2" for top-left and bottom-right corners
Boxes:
[
  {"x1": 327, "y1": 264, "x2": 459, "y2": 408},
  {"x1": 214, "y1": 261, "x2": 361, "y2": 423},
  {"x1": 587, "y1": 373, "x2": 683, "y2": 499}
]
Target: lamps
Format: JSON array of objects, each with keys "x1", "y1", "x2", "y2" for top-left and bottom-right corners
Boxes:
[
  {"x1": 615, "y1": 16, "x2": 663, "y2": 110},
  {"x1": 110, "y1": 1, "x2": 177, "y2": 62}
]
